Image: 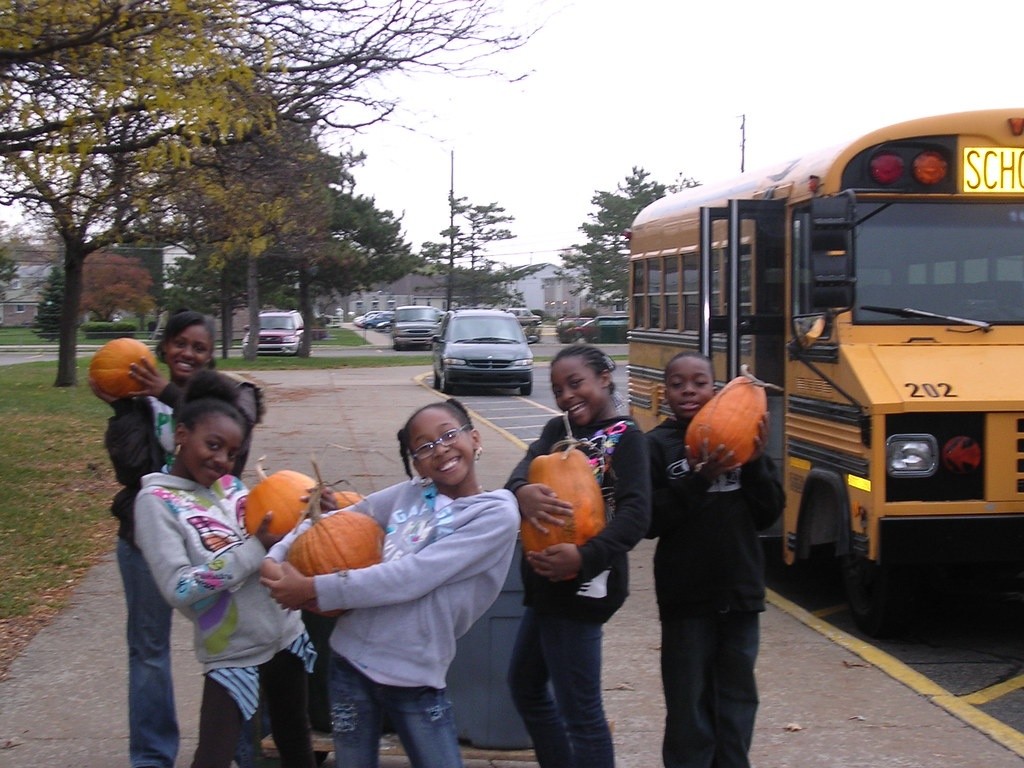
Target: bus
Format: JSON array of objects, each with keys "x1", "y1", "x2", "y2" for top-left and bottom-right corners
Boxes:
[{"x1": 625, "y1": 107, "x2": 1024, "y2": 611}]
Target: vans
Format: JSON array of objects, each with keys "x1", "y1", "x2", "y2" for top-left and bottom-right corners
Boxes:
[{"x1": 431, "y1": 309, "x2": 534, "y2": 396}]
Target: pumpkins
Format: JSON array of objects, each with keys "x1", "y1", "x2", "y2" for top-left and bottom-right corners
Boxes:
[
  {"x1": 245, "y1": 455, "x2": 320, "y2": 537},
  {"x1": 684, "y1": 365, "x2": 784, "y2": 468},
  {"x1": 330, "y1": 490, "x2": 367, "y2": 510},
  {"x1": 90, "y1": 337, "x2": 155, "y2": 398},
  {"x1": 522, "y1": 410, "x2": 608, "y2": 580},
  {"x1": 287, "y1": 489, "x2": 387, "y2": 616}
]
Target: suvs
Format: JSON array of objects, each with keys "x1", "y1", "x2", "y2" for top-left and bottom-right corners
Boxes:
[
  {"x1": 391, "y1": 305, "x2": 443, "y2": 351},
  {"x1": 503, "y1": 308, "x2": 542, "y2": 326},
  {"x1": 241, "y1": 308, "x2": 304, "y2": 356}
]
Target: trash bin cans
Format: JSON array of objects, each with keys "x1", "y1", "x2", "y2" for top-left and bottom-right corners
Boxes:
[{"x1": 448, "y1": 545, "x2": 538, "y2": 753}]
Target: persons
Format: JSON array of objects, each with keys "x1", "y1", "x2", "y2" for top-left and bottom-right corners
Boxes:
[
  {"x1": 504, "y1": 345, "x2": 651, "y2": 768},
  {"x1": 132, "y1": 397, "x2": 320, "y2": 768},
  {"x1": 261, "y1": 398, "x2": 521, "y2": 768},
  {"x1": 642, "y1": 351, "x2": 786, "y2": 768},
  {"x1": 86, "y1": 314, "x2": 263, "y2": 768}
]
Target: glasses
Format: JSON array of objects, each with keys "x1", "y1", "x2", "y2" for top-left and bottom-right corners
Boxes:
[{"x1": 407, "y1": 423, "x2": 474, "y2": 461}]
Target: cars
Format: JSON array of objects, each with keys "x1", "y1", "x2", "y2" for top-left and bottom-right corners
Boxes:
[
  {"x1": 375, "y1": 320, "x2": 393, "y2": 333},
  {"x1": 556, "y1": 316, "x2": 628, "y2": 344},
  {"x1": 353, "y1": 311, "x2": 394, "y2": 329}
]
